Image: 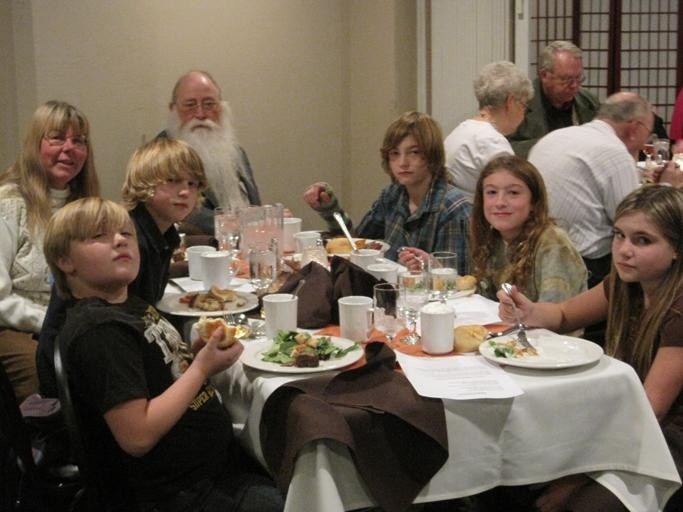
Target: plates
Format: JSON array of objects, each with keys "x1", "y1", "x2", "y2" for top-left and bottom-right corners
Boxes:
[
  {"x1": 479, "y1": 333, "x2": 602, "y2": 371},
  {"x1": 239, "y1": 334, "x2": 364, "y2": 373},
  {"x1": 153, "y1": 288, "x2": 258, "y2": 316}
]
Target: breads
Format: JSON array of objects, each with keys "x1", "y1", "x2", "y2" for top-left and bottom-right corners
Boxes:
[
  {"x1": 189, "y1": 286, "x2": 245, "y2": 311},
  {"x1": 453, "y1": 324, "x2": 490, "y2": 352},
  {"x1": 197, "y1": 318, "x2": 236, "y2": 348},
  {"x1": 326, "y1": 238, "x2": 351, "y2": 253},
  {"x1": 456, "y1": 275, "x2": 476, "y2": 291}
]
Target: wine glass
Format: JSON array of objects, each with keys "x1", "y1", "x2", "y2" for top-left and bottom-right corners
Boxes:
[
  {"x1": 373, "y1": 283, "x2": 409, "y2": 352},
  {"x1": 638, "y1": 132, "x2": 682, "y2": 172}
]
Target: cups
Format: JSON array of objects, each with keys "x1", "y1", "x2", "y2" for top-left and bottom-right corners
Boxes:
[
  {"x1": 261, "y1": 294, "x2": 298, "y2": 343},
  {"x1": 350, "y1": 250, "x2": 475, "y2": 299},
  {"x1": 172, "y1": 203, "x2": 328, "y2": 287},
  {"x1": 336, "y1": 296, "x2": 378, "y2": 345}
]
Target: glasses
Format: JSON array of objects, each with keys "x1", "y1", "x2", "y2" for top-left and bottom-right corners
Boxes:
[
  {"x1": 42, "y1": 135, "x2": 87, "y2": 148},
  {"x1": 546, "y1": 68, "x2": 585, "y2": 85}
]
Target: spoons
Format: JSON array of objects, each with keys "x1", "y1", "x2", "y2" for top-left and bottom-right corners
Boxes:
[{"x1": 234, "y1": 314, "x2": 252, "y2": 328}]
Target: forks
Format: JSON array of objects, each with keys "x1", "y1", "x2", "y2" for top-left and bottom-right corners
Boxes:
[
  {"x1": 222, "y1": 312, "x2": 236, "y2": 327},
  {"x1": 501, "y1": 281, "x2": 534, "y2": 350}
]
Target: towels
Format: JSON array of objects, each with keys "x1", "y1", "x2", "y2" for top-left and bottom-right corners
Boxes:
[
  {"x1": 255, "y1": 338, "x2": 449, "y2": 512},
  {"x1": 240, "y1": 250, "x2": 397, "y2": 334}
]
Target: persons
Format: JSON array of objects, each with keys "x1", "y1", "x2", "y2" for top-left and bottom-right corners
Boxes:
[
  {"x1": 43, "y1": 195, "x2": 282, "y2": 512},
  {"x1": 440, "y1": 57, "x2": 536, "y2": 191},
  {"x1": 0, "y1": 101, "x2": 100, "y2": 435},
  {"x1": 41, "y1": 136, "x2": 206, "y2": 362},
  {"x1": 670, "y1": 85, "x2": 683, "y2": 154},
  {"x1": 505, "y1": 37, "x2": 602, "y2": 152},
  {"x1": 395, "y1": 154, "x2": 591, "y2": 345},
  {"x1": 498, "y1": 183, "x2": 682, "y2": 512},
  {"x1": 303, "y1": 111, "x2": 474, "y2": 276},
  {"x1": 158, "y1": 71, "x2": 263, "y2": 249},
  {"x1": 520, "y1": 92, "x2": 656, "y2": 284}
]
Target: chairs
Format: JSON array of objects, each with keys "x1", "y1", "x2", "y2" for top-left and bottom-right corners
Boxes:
[
  {"x1": 0, "y1": 363, "x2": 71, "y2": 510},
  {"x1": 48, "y1": 329, "x2": 108, "y2": 510}
]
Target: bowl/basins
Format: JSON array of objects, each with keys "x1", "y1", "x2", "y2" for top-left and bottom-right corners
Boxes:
[{"x1": 324, "y1": 237, "x2": 391, "y2": 266}]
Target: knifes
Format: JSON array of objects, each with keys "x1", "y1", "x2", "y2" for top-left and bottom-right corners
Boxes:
[{"x1": 485, "y1": 319, "x2": 521, "y2": 339}]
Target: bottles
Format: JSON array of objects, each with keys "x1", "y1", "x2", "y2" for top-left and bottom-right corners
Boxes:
[{"x1": 418, "y1": 291, "x2": 456, "y2": 355}]
